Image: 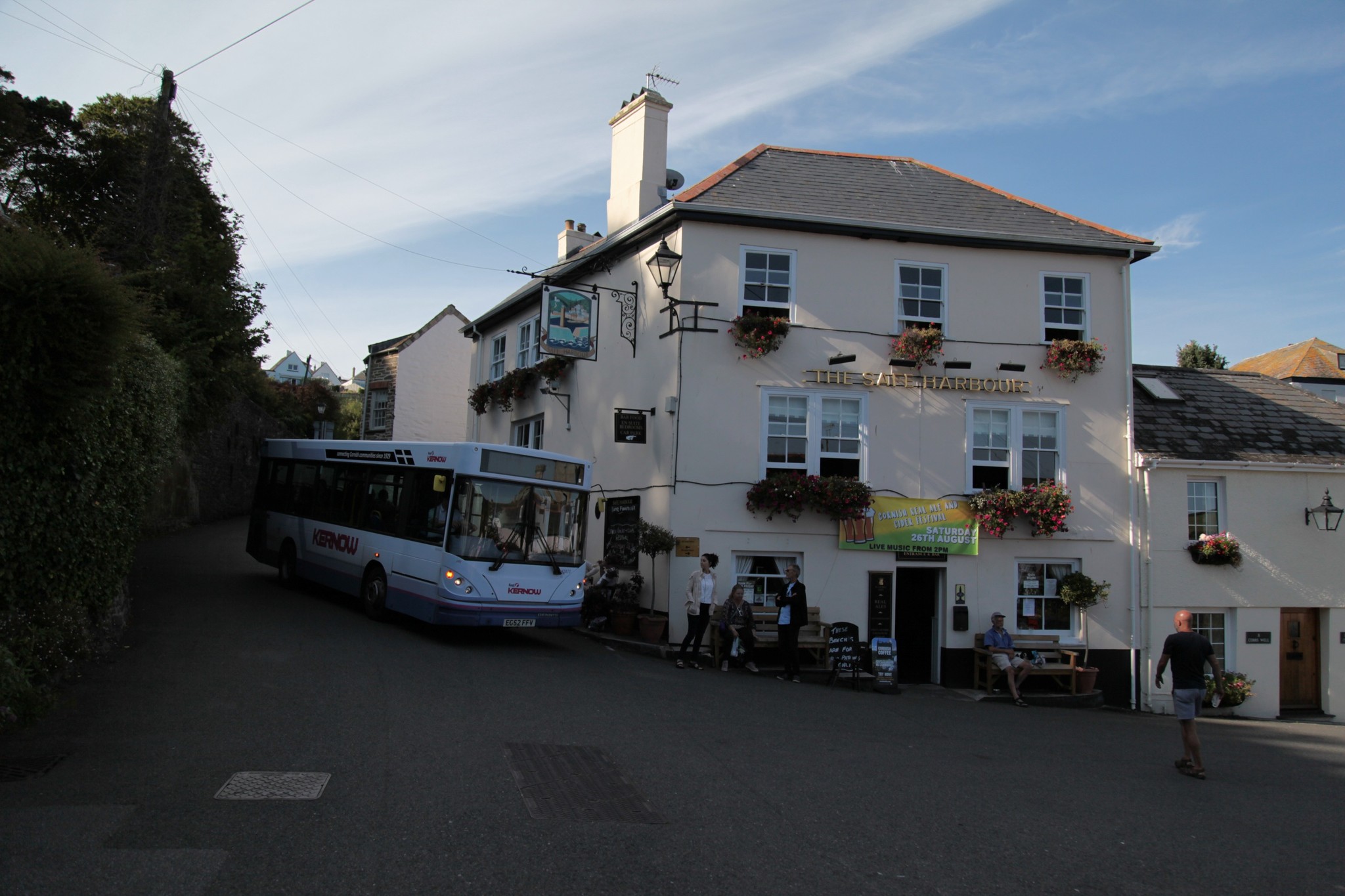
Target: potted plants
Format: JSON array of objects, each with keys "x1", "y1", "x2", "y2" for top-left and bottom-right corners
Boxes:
[
  {"x1": 634, "y1": 518, "x2": 680, "y2": 641},
  {"x1": 609, "y1": 569, "x2": 644, "y2": 630},
  {"x1": 584, "y1": 583, "x2": 614, "y2": 630},
  {"x1": 1057, "y1": 570, "x2": 1112, "y2": 691}
]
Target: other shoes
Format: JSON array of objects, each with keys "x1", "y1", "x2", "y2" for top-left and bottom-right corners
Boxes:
[
  {"x1": 792, "y1": 675, "x2": 800, "y2": 682},
  {"x1": 745, "y1": 662, "x2": 759, "y2": 672},
  {"x1": 721, "y1": 660, "x2": 728, "y2": 671},
  {"x1": 776, "y1": 674, "x2": 794, "y2": 680}
]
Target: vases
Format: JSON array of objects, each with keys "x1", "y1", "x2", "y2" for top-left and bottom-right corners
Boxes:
[
  {"x1": 1222, "y1": 694, "x2": 1246, "y2": 707},
  {"x1": 1191, "y1": 550, "x2": 1230, "y2": 565},
  {"x1": 499, "y1": 387, "x2": 511, "y2": 399},
  {"x1": 513, "y1": 385, "x2": 524, "y2": 397},
  {"x1": 1202, "y1": 694, "x2": 1216, "y2": 709}
]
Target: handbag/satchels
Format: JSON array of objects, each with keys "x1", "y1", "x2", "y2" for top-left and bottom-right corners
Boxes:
[
  {"x1": 730, "y1": 636, "x2": 740, "y2": 657},
  {"x1": 1013, "y1": 648, "x2": 1046, "y2": 664}
]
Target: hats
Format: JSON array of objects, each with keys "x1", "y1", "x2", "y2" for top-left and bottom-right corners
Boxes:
[{"x1": 991, "y1": 612, "x2": 1006, "y2": 620}]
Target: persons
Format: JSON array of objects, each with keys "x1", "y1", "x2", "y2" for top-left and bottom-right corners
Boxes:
[
  {"x1": 377, "y1": 489, "x2": 394, "y2": 506},
  {"x1": 775, "y1": 564, "x2": 808, "y2": 683},
  {"x1": 675, "y1": 553, "x2": 719, "y2": 670},
  {"x1": 721, "y1": 584, "x2": 759, "y2": 672},
  {"x1": 428, "y1": 492, "x2": 477, "y2": 534},
  {"x1": 983, "y1": 612, "x2": 1031, "y2": 707},
  {"x1": 366, "y1": 490, "x2": 376, "y2": 505},
  {"x1": 583, "y1": 560, "x2": 600, "y2": 581},
  {"x1": 1155, "y1": 610, "x2": 1225, "y2": 779}
]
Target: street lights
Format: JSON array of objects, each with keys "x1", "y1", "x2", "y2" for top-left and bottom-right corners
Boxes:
[{"x1": 317, "y1": 400, "x2": 326, "y2": 439}]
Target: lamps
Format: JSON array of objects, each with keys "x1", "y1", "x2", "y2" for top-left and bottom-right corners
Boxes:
[
  {"x1": 1304, "y1": 487, "x2": 1343, "y2": 531},
  {"x1": 646, "y1": 232, "x2": 683, "y2": 315}
]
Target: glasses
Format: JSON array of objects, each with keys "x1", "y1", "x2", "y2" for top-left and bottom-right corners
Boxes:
[
  {"x1": 785, "y1": 569, "x2": 795, "y2": 573},
  {"x1": 737, "y1": 607, "x2": 743, "y2": 616}
]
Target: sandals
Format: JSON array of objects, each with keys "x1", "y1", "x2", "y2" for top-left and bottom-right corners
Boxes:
[
  {"x1": 1012, "y1": 696, "x2": 1028, "y2": 707},
  {"x1": 689, "y1": 662, "x2": 704, "y2": 670},
  {"x1": 1015, "y1": 688, "x2": 1025, "y2": 697},
  {"x1": 676, "y1": 659, "x2": 684, "y2": 669}
]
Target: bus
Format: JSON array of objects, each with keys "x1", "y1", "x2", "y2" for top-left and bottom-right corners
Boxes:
[{"x1": 245, "y1": 437, "x2": 607, "y2": 631}]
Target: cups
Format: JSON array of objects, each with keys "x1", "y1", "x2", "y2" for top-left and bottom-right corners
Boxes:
[{"x1": 1212, "y1": 693, "x2": 1221, "y2": 707}]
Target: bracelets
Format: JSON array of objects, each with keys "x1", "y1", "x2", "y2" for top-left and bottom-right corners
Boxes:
[{"x1": 1156, "y1": 674, "x2": 1162, "y2": 679}]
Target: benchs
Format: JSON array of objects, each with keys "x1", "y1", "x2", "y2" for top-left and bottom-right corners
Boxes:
[
  {"x1": 972, "y1": 633, "x2": 1079, "y2": 695},
  {"x1": 708, "y1": 605, "x2": 833, "y2": 680}
]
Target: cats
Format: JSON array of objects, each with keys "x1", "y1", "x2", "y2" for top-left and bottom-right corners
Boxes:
[{"x1": 1016, "y1": 650, "x2": 1045, "y2": 668}]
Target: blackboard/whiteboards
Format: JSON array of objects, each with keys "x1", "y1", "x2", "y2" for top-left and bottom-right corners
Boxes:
[
  {"x1": 603, "y1": 495, "x2": 640, "y2": 571},
  {"x1": 823, "y1": 622, "x2": 860, "y2": 672}
]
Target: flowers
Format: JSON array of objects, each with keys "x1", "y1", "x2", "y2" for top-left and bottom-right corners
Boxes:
[
  {"x1": 968, "y1": 479, "x2": 1074, "y2": 538},
  {"x1": 746, "y1": 471, "x2": 875, "y2": 525},
  {"x1": 727, "y1": 308, "x2": 790, "y2": 360},
  {"x1": 1204, "y1": 670, "x2": 1259, "y2": 703},
  {"x1": 1040, "y1": 338, "x2": 1106, "y2": 384},
  {"x1": 467, "y1": 356, "x2": 567, "y2": 417},
  {"x1": 1183, "y1": 530, "x2": 1244, "y2": 574},
  {"x1": 886, "y1": 322, "x2": 945, "y2": 369}
]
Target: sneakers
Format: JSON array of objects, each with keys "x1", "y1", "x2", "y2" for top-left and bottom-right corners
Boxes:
[
  {"x1": 1175, "y1": 757, "x2": 1193, "y2": 768},
  {"x1": 1179, "y1": 766, "x2": 1205, "y2": 780}
]
